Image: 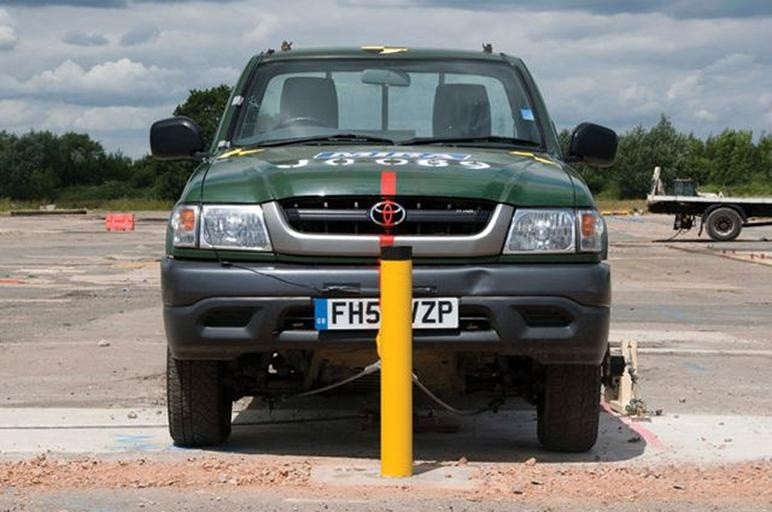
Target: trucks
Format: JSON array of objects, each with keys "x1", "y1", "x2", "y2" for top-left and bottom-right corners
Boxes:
[{"x1": 645, "y1": 164, "x2": 772, "y2": 240}]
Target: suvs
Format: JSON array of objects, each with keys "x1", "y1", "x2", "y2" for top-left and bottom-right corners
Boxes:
[{"x1": 148, "y1": 34, "x2": 620, "y2": 449}]
